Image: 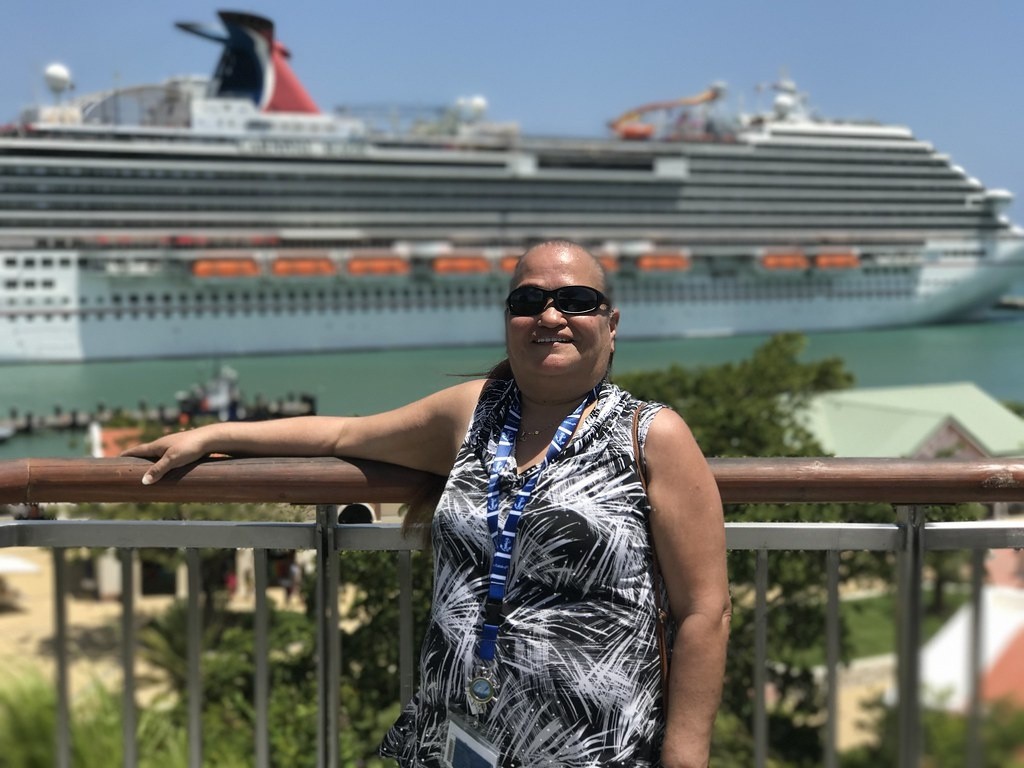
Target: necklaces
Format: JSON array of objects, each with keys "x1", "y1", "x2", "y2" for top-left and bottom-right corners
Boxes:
[{"x1": 518, "y1": 399, "x2": 586, "y2": 441}]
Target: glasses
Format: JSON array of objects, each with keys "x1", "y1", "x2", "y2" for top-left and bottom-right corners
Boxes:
[{"x1": 505, "y1": 286, "x2": 611, "y2": 315}]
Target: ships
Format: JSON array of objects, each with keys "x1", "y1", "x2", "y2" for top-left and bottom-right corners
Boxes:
[{"x1": 2, "y1": 9, "x2": 1024, "y2": 368}]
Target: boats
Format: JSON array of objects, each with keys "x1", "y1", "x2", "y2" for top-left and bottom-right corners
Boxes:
[{"x1": 192, "y1": 252, "x2": 870, "y2": 279}]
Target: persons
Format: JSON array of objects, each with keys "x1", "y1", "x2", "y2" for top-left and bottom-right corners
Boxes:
[{"x1": 120, "y1": 239, "x2": 731, "y2": 767}]
